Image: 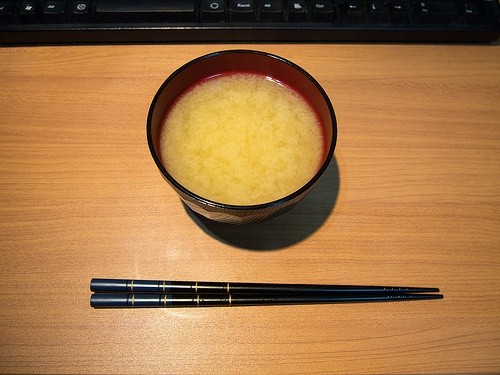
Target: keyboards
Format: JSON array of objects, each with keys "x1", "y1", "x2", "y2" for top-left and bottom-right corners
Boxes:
[{"x1": 0, "y1": 0, "x2": 500, "y2": 45}]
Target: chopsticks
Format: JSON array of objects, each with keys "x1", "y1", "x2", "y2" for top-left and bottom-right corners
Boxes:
[{"x1": 89, "y1": 277, "x2": 444, "y2": 308}]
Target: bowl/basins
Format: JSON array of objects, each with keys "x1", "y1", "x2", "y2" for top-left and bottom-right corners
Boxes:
[{"x1": 147, "y1": 50, "x2": 338, "y2": 225}]
